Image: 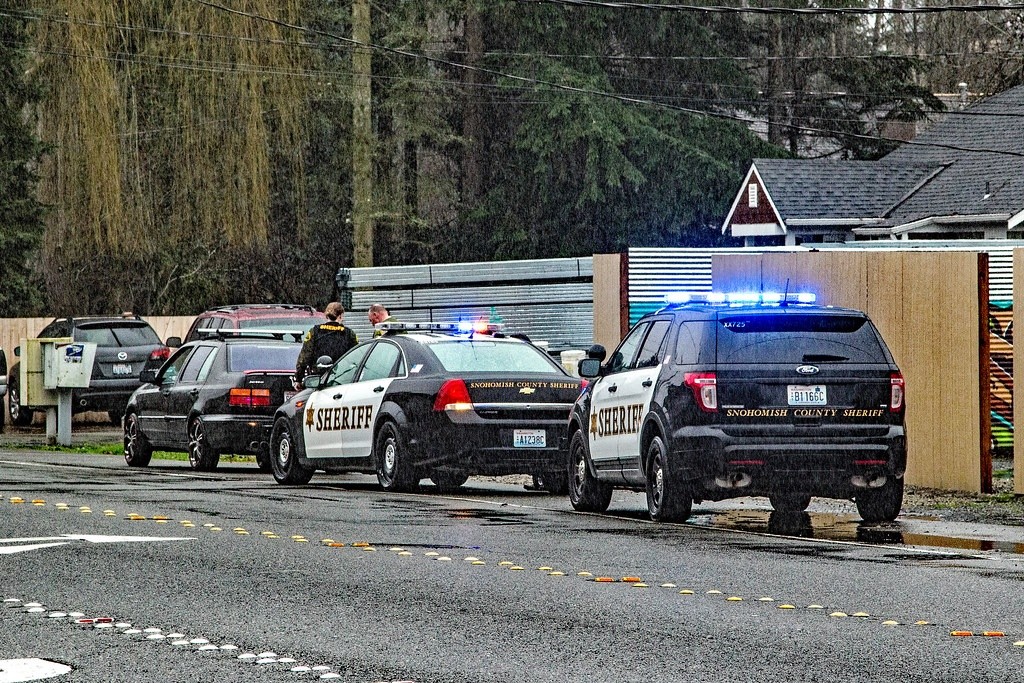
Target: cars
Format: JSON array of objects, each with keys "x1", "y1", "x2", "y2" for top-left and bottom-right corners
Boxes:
[
  {"x1": 121, "y1": 329, "x2": 306, "y2": 473},
  {"x1": 269, "y1": 321, "x2": 590, "y2": 494}
]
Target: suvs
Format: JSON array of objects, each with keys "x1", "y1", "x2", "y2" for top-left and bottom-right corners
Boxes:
[
  {"x1": 7, "y1": 314, "x2": 172, "y2": 428},
  {"x1": 166, "y1": 303, "x2": 331, "y2": 349},
  {"x1": 0, "y1": 347, "x2": 8, "y2": 433},
  {"x1": 564, "y1": 292, "x2": 906, "y2": 525}
]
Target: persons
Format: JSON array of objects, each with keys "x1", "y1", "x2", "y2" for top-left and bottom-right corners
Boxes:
[
  {"x1": 368, "y1": 304, "x2": 408, "y2": 339},
  {"x1": 294, "y1": 302, "x2": 359, "y2": 393}
]
